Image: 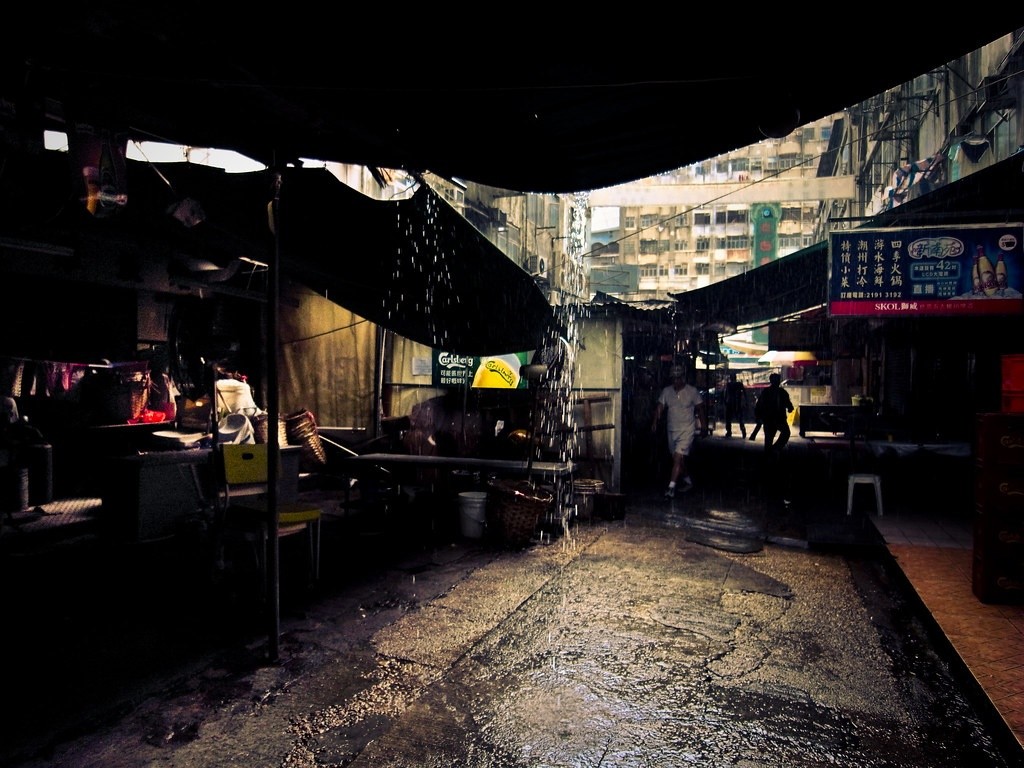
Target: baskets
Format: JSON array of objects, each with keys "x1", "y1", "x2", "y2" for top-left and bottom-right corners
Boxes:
[
  {"x1": 489, "y1": 478, "x2": 554, "y2": 540},
  {"x1": 107, "y1": 361, "x2": 153, "y2": 420},
  {"x1": 253, "y1": 410, "x2": 327, "y2": 467}
]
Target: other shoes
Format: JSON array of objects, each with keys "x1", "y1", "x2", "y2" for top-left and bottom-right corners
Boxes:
[
  {"x1": 742, "y1": 432, "x2": 746, "y2": 439},
  {"x1": 664, "y1": 487, "x2": 676, "y2": 498},
  {"x1": 679, "y1": 483, "x2": 693, "y2": 492},
  {"x1": 748, "y1": 435, "x2": 755, "y2": 441},
  {"x1": 726, "y1": 432, "x2": 732, "y2": 437}
]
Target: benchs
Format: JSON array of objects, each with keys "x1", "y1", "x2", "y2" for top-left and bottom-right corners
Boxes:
[{"x1": 343, "y1": 389, "x2": 577, "y2": 475}]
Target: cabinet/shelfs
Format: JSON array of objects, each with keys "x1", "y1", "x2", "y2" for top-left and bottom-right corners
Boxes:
[{"x1": 972, "y1": 412, "x2": 1024, "y2": 608}]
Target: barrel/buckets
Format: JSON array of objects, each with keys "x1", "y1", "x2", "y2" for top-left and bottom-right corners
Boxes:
[{"x1": 458, "y1": 491, "x2": 488, "y2": 539}]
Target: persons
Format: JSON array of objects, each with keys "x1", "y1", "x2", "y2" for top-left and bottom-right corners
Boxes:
[
  {"x1": 723, "y1": 373, "x2": 749, "y2": 439},
  {"x1": 758, "y1": 374, "x2": 793, "y2": 452},
  {"x1": 651, "y1": 365, "x2": 708, "y2": 499},
  {"x1": 0, "y1": 396, "x2": 47, "y2": 507}
]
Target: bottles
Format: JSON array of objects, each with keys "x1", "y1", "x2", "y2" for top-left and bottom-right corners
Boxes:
[{"x1": 970, "y1": 245, "x2": 1008, "y2": 298}]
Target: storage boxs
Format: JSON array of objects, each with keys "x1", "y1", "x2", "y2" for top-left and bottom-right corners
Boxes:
[
  {"x1": 593, "y1": 491, "x2": 627, "y2": 522},
  {"x1": 851, "y1": 394, "x2": 874, "y2": 405},
  {"x1": 999, "y1": 353, "x2": 1024, "y2": 412}
]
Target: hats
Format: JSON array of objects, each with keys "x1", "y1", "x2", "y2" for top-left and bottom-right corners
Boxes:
[{"x1": 669, "y1": 365, "x2": 684, "y2": 378}]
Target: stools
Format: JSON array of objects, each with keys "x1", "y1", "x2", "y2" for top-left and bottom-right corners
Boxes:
[{"x1": 846, "y1": 474, "x2": 884, "y2": 517}]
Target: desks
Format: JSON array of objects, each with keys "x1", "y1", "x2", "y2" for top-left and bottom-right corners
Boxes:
[{"x1": 799, "y1": 404, "x2": 873, "y2": 438}]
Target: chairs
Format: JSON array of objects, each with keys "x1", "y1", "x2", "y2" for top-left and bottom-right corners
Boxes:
[{"x1": 220, "y1": 443, "x2": 325, "y2": 578}]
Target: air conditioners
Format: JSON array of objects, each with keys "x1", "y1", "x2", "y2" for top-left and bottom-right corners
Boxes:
[
  {"x1": 975, "y1": 77, "x2": 1003, "y2": 114},
  {"x1": 528, "y1": 255, "x2": 548, "y2": 280},
  {"x1": 489, "y1": 208, "x2": 507, "y2": 227}
]
